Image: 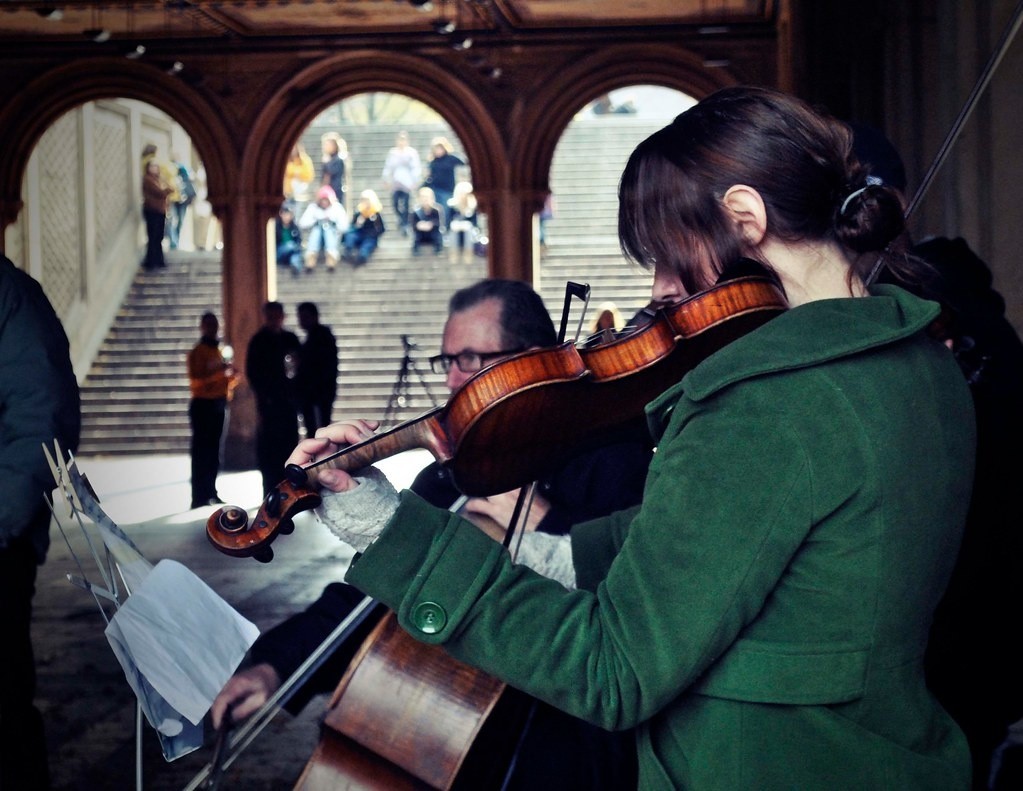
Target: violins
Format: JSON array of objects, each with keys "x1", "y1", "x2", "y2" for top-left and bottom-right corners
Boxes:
[{"x1": 205, "y1": 272, "x2": 790, "y2": 563}]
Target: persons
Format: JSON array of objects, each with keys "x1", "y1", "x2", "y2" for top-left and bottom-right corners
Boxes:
[
  {"x1": 206, "y1": 277, "x2": 655, "y2": 790},
  {"x1": 291, "y1": 300, "x2": 339, "y2": 445},
  {"x1": 838, "y1": 111, "x2": 1023, "y2": 790},
  {"x1": 283, "y1": 87, "x2": 980, "y2": 790},
  {"x1": 137, "y1": 130, "x2": 555, "y2": 279},
  {"x1": 0, "y1": 105, "x2": 83, "y2": 790},
  {"x1": 187, "y1": 310, "x2": 238, "y2": 509},
  {"x1": 245, "y1": 303, "x2": 305, "y2": 505}
]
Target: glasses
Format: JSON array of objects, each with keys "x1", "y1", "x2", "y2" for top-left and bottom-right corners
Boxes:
[{"x1": 432, "y1": 348, "x2": 524, "y2": 374}]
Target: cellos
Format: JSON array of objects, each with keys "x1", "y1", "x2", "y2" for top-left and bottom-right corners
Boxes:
[{"x1": 286, "y1": 487, "x2": 564, "y2": 791}]
